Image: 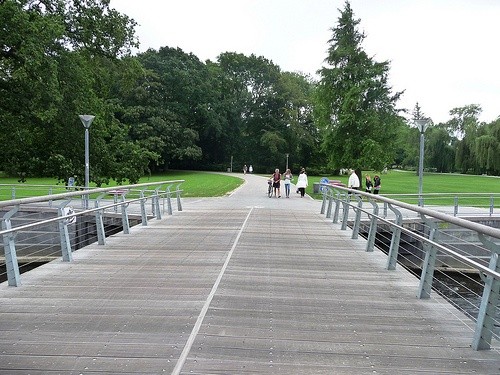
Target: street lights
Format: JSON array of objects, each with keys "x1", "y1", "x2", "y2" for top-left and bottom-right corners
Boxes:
[
  {"x1": 78, "y1": 115, "x2": 95, "y2": 208},
  {"x1": 285, "y1": 153, "x2": 290, "y2": 169},
  {"x1": 413, "y1": 119, "x2": 431, "y2": 208}
]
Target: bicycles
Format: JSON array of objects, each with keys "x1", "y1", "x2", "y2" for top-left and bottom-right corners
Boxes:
[{"x1": 267, "y1": 179, "x2": 276, "y2": 198}]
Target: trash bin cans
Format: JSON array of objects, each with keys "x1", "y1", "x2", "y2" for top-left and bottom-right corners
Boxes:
[
  {"x1": 227, "y1": 168, "x2": 231, "y2": 173},
  {"x1": 313, "y1": 184, "x2": 319, "y2": 193}
]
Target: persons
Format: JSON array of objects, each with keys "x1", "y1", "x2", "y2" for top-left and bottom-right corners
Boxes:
[
  {"x1": 283, "y1": 169, "x2": 293, "y2": 198},
  {"x1": 346, "y1": 170, "x2": 362, "y2": 203},
  {"x1": 249, "y1": 165, "x2": 253, "y2": 174},
  {"x1": 366, "y1": 174, "x2": 381, "y2": 194},
  {"x1": 296, "y1": 168, "x2": 309, "y2": 198},
  {"x1": 243, "y1": 164, "x2": 248, "y2": 175},
  {"x1": 270, "y1": 168, "x2": 281, "y2": 198}
]
